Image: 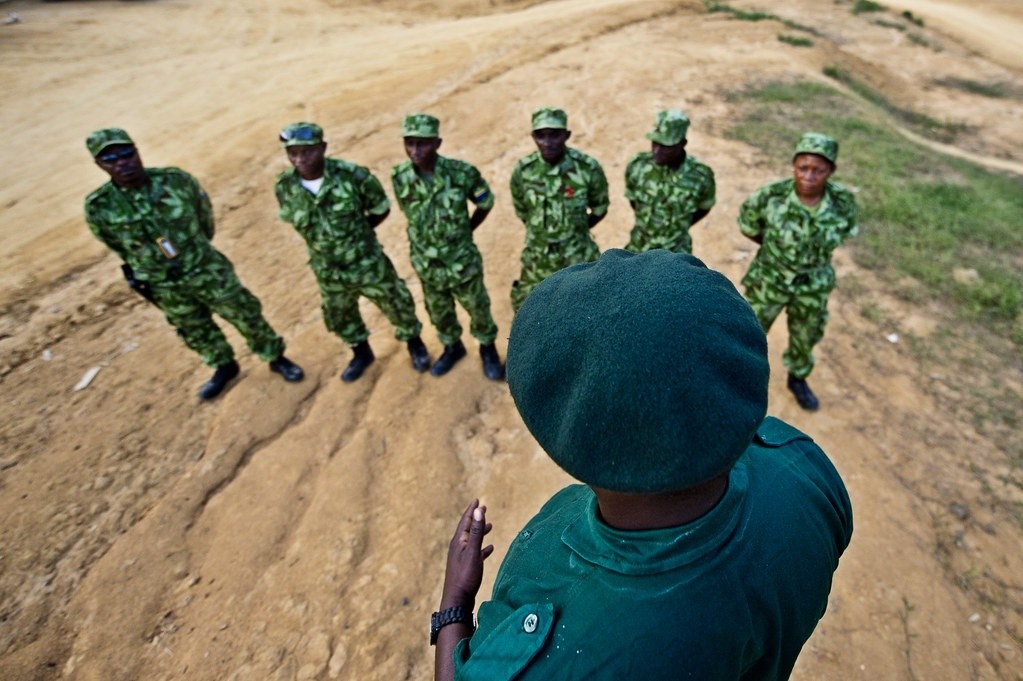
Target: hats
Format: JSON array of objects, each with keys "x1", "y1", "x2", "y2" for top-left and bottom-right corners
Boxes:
[
  {"x1": 504, "y1": 248, "x2": 771, "y2": 493},
  {"x1": 279, "y1": 122, "x2": 323, "y2": 148},
  {"x1": 792, "y1": 131, "x2": 838, "y2": 167},
  {"x1": 403, "y1": 114, "x2": 440, "y2": 138},
  {"x1": 647, "y1": 112, "x2": 691, "y2": 146},
  {"x1": 86, "y1": 130, "x2": 135, "y2": 158},
  {"x1": 531, "y1": 108, "x2": 567, "y2": 131}
]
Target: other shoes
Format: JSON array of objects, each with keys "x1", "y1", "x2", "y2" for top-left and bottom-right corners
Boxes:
[
  {"x1": 407, "y1": 336, "x2": 431, "y2": 374},
  {"x1": 430, "y1": 339, "x2": 467, "y2": 378},
  {"x1": 270, "y1": 355, "x2": 304, "y2": 382},
  {"x1": 341, "y1": 339, "x2": 375, "y2": 381},
  {"x1": 197, "y1": 359, "x2": 239, "y2": 400},
  {"x1": 788, "y1": 373, "x2": 819, "y2": 412},
  {"x1": 479, "y1": 343, "x2": 505, "y2": 380}
]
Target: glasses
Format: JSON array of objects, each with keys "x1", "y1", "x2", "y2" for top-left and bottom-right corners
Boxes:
[
  {"x1": 99, "y1": 147, "x2": 136, "y2": 163},
  {"x1": 279, "y1": 127, "x2": 316, "y2": 141}
]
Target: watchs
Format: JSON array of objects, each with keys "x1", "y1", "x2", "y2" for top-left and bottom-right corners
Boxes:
[{"x1": 429, "y1": 605, "x2": 477, "y2": 646}]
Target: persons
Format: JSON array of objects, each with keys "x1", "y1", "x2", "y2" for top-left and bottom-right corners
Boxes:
[
  {"x1": 735, "y1": 134, "x2": 857, "y2": 410},
  {"x1": 391, "y1": 114, "x2": 507, "y2": 380},
  {"x1": 425, "y1": 248, "x2": 854, "y2": 681},
  {"x1": 621, "y1": 109, "x2": 716, "y2": 256},
  {"x1": 275, "y1": 122, "x2": 431, "y2": 381},
  {"x1": 509, "y1": 107, "x2": 610, "y2": 314},
  {"x1": 82, "y1": 128, "x2": 306, "y2": 401}
]
